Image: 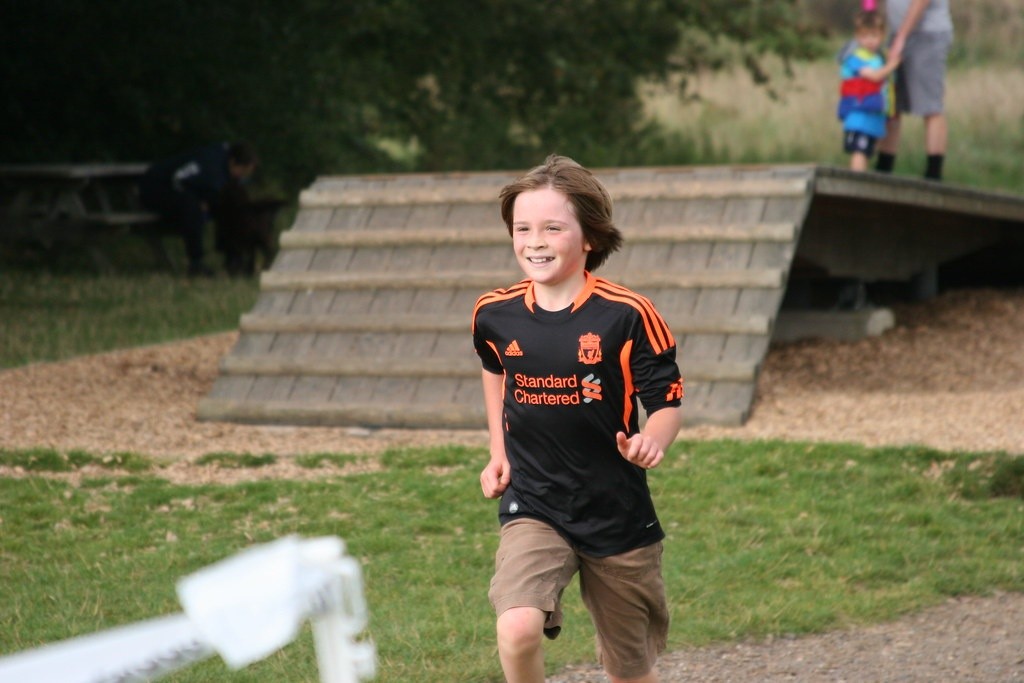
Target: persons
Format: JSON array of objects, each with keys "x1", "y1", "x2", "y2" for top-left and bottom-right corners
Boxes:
[
  {"x1": 471, "y1": 155, "x2": 684, "y2": 683},
  {"x1": 130, "y1": 138, "x2": 264, "y2": 275},
  {"x1": 875, "y1": 0, "x2": 954, "y2": 183},
  {"x1": 838, "y1": 10, "x2": 903, "y2": 172}
]
